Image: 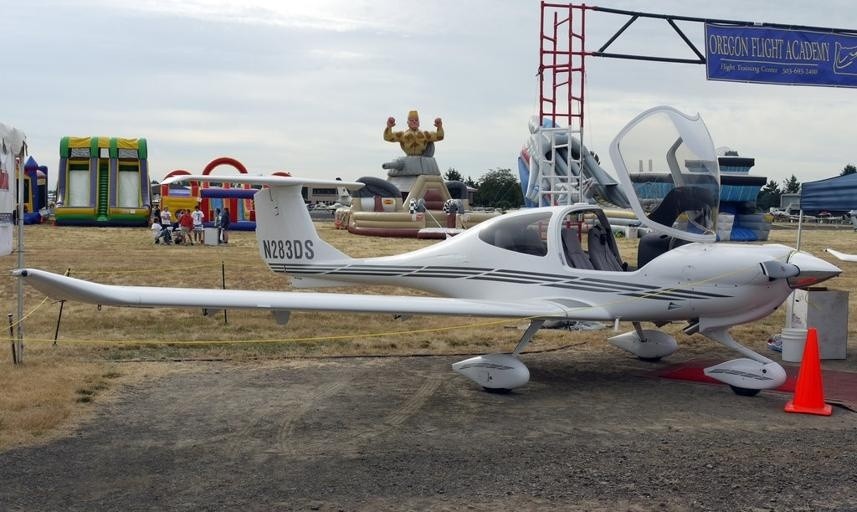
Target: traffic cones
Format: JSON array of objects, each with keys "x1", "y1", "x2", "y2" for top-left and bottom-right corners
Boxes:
[{"x1": 783, "y1": 327, "x2": 833, "y2": 416}]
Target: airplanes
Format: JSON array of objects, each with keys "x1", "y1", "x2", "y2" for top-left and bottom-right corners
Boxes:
[{"x1": 9, "y1": 104, "x2": 844, "y2": 396}]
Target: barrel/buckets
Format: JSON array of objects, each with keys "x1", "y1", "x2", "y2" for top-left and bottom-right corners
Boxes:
[{"x1": 781, "y1": 328, "x2": 807, "y2": 363}]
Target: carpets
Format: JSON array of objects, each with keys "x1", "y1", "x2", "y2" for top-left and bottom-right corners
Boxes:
[{"x1": 638, "y1": 354, "x2": 856, "y2": 411}]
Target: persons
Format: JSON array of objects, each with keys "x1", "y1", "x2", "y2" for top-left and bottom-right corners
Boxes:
[
  {"x1": 151, "y1": 205, "x2": 230, "y2": 246},
  {"x1": 384, "y1": 111, "x2": 444, "y2": 155}
]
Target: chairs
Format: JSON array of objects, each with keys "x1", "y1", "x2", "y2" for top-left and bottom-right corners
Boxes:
[{"x1": 491, "y1": 221, "x2": 624, "y2": 274}]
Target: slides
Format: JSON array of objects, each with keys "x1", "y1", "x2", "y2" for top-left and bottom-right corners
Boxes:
[
  {"x1": 209, "y1": 185, "x2": 247, "y2": 223},
  {"x1": 65, "y1": 170, "x2": 143, "y2": 221}
]
